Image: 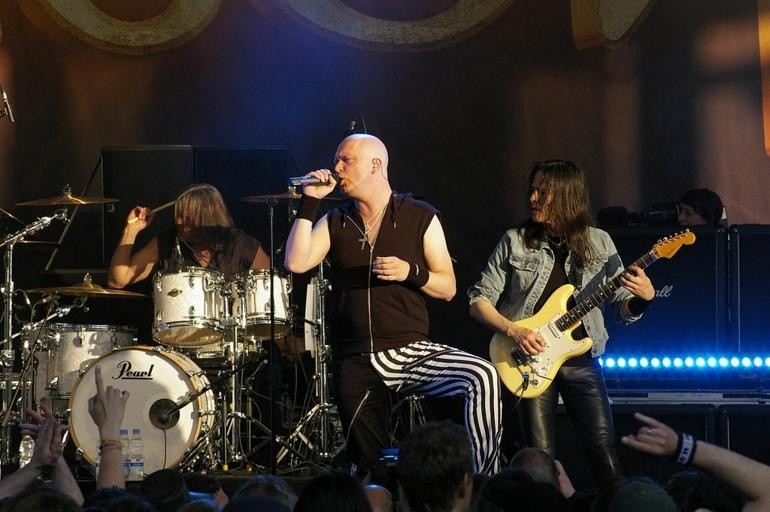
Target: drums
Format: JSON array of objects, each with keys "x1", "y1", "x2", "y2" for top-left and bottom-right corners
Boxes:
[
  {"x1": 22, "y1": 322, "x2": 138, "y2": 425},
  {"x1": 152, "y1": 265, "x2": 228, "y2": 350},
  {"x1": 226, "y1": 270, "x2": 294, "y2": 341},
  {"x1": 67, "y1": 344, "x2": 216, "y2": 483}
]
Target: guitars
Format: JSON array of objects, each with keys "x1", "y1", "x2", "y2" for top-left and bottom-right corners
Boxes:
[{"x1": 487, "y1": 227, "x2": 697, "y2": 400}]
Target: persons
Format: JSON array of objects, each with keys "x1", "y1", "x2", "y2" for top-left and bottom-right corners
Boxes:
[
  {"x1": 508, "y1": 446, "x2": 587, "y2": 510},
  {"x1": 234, "y1": 475, "x2": 298, "y2": 512},
  {"x1": 574, "y1": 487, "x2": 592, "y2": 512},
  {"x1": 663, "y1": 464, "x2": 709, "y2": 511},
  {"x1": 108, "y1": 184, "x2": 272, "y2": 293},
  {"x1": 222, "y1": 496, "x2": 290, "y2": 512},
  {"x1": 292, "y1": 471, "x2": 373, "y2": 512},
  {"x1": 477, "y1": 469, "x2": 537, "y2": 510},
  {"x1": 0, "y1": 397, "x2": 86, "y2": 511},
  {"x1": 675, "y1": 187, "x2": 726, "y2": 228},
  {"x1": 503, "y1": 481, "x2": 570, "y2": 512},
  {"x1": 606, "y1": 410, "x2": 770, "y2": 512},
  {"x1": 137, "y1": 467, "x2": 192, "y2": 512},
  {"x1": 181, "y1": 487, "x2": 228, "y2": 511},
  {"x1": 687, "y1": 478, "x2": 744, "y2": 512},
  {"x1": 467, "y1": 156, "x2": 654, "y2": 486},
  {"x1": 473, "y1": 471, "x2": 492, "y2": 510},
  {"x1": 394, "y1": 416, "x2": 475, "y2": 511},
  {"x1": 363, "y1": 484, "x2": 394, "y2": 512},
  {"x1": 283, "y1": 132, "x2": 504, "y2": 476},
  {"x1": 87, "y1": 363, "x2": 153, "y2": 512}
]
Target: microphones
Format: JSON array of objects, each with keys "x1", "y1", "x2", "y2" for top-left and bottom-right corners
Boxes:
[
  {"x1": 288, "y1": 175, "x2": 340, "y2": 187},
  {"x1": 0, "y1": 87, "x2": 15, "y2": 123}
]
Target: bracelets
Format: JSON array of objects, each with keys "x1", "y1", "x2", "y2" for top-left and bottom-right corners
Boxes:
[{"x1": 671, "y1": 430, "x2": 696, "y2": 467}]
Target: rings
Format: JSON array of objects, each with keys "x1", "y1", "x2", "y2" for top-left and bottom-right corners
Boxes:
[{"x1": 648, "y1": 426, "x2": 654, "y2": 436}]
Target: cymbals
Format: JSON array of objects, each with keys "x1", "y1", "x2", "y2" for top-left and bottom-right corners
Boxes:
[
  {"x1": 25, "y1": 273, "x2": 147, "y2": 297},
  {"x1": 240, "y1": 186, "x2": 343, "y2": 203},
  {"x1": 14, "y1": 185, "x2": 120, "y2": 205}
]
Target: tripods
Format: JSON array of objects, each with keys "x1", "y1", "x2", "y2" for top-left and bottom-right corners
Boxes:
[
  {"x1": 184, "y1": 329, "x2": 307, "y2": 471},
  {"x1": 272, "y1": 262, "x2": 343, "y2": 470}
]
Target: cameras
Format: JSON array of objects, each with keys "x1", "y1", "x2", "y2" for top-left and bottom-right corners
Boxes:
[{"x1": 598, "y1": 200, "x2": 681, "y2": 227}]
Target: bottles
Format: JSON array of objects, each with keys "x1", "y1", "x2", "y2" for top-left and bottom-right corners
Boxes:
[
  {"x1": 19, "y1": 431, "x2": 35, "y2": 469},
  {"x1": 95, "y1": 438, "x2": 101, "y2": 486},
  {"x1": 118, "y1": 429, "x2": 130, "y2": 482},
  {"x1": 129, "y1": 429, "x2": 143, "y2": 482}
]
